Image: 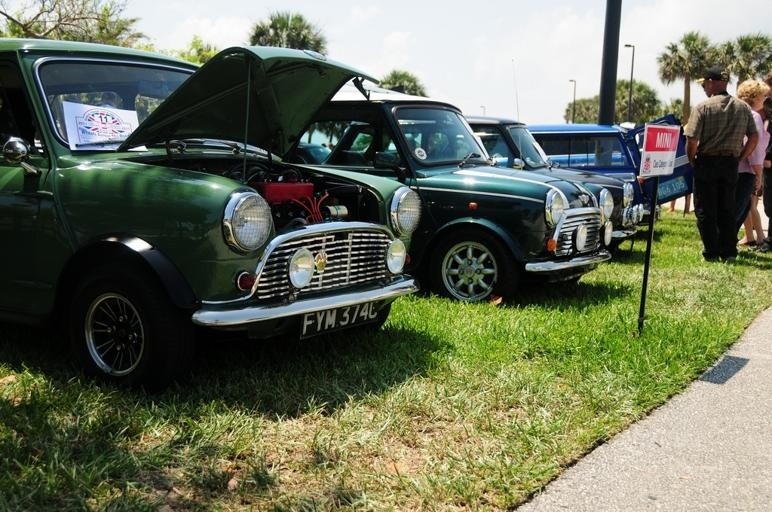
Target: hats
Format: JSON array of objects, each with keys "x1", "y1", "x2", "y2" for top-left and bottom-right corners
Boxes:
[{"x1": 705, "y1": 66, "x2": 730, "y2": 82}]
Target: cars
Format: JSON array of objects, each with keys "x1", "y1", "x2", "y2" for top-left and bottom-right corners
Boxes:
[{"x1": 0, "y1": 37, "x2": 423, "y2": 390}]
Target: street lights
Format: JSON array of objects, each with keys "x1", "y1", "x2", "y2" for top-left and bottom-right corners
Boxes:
[
  {"x1": 569, "y1": 79, "x2": 577, "y2": 124},
  {"x1": 625, "y1": 44, "x2": 635, "y2": 122}
]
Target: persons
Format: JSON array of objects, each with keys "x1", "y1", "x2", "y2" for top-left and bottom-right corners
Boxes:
[
  {"x1": 736, "y1": 79, "x2": 771, "y2": 246},
  {"x1": 762, "y1": 118, "x2": 772, "y2": 247},
  {"x1": 739, "y1": 190, "x2": 765, "y2": 245},
  {"x1": 683, "y1": 67, "x2": 759, "y2": 262}
]
